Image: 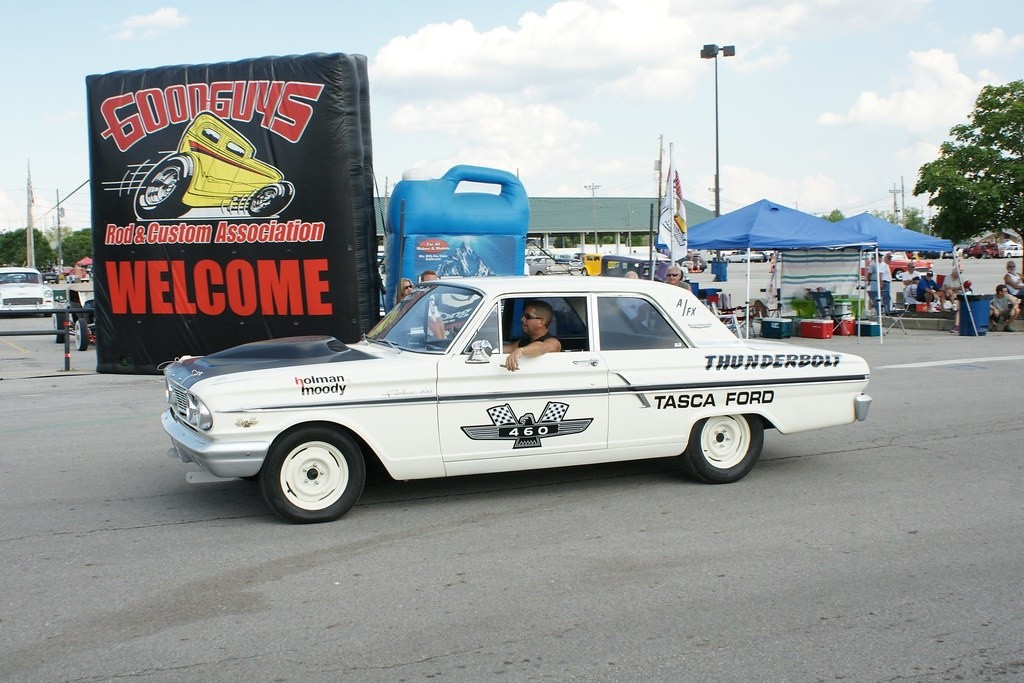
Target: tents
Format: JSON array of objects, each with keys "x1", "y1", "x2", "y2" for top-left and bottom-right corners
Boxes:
[
  {"x1": 772, "y1": 210, "x2": 979, "y2": 343},
  {"x1": 77, "y1": 257, "x2": 93, "y2": 268},
  {"x1": 650, "y1": 198, "x2": 885, "y2": 346}
]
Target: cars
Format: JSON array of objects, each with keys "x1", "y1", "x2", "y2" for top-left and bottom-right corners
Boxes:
[
  {"x1": 1, "y1": 262, "x2": 96, "y2": 351},
  {"x1": 157, "y1": 272, "x2": 872, "y2": 527},
  {"x1": 859, "y1": 238, "x2": 1024, "y2": 283},
  {"x1": 522, "y1": 248, "x2": 782, "y2": 282}
]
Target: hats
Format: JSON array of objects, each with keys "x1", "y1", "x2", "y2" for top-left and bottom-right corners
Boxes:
[
  {"x1": 874, "y1": 252, "x2": 883, "y2": 256},
  {"x1": 927, "y1": 270, "x2": 933, "y2": 276},
  {"x1": 884, "y1": 252, "x2": 892, "y2": 258}
]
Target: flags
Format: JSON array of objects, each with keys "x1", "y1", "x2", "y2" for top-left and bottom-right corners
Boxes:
[{"x1": 654, "y1": 159, "x2": 688, "y2": 262}]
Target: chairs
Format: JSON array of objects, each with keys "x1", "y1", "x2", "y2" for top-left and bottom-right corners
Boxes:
[
  {"x1": 896, "y1": 274, "x2": 974, "y2": 312},
  {"x1": 810, "y1": 291, "x2": 853, "y2": 336},
  {"x1": 698, "y1": 291, "x2": 778, "y2": 338},
  {"x1": 866, "y1": 290, "x2": 916, "y2": 335}
]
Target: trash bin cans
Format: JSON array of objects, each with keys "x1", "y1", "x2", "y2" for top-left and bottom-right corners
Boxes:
[
  {"x1": 956, "y1": 294, "x2": 993, "y2": 336},
  {"x1": 711, "y1": 257, "x2": 716, "y2": 274},
  {"x1": 716, "y1": 261, "x2": 727, "y2": 282}
]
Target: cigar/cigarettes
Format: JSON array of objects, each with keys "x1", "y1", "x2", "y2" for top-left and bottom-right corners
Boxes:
[{"x1": 499, "y1": 364, "x2": 520, "y2": 370}]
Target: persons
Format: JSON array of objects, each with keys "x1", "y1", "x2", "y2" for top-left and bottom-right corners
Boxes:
[
  {"x1": 989, "y1": 285, "x2": 1020, "y2": 333},
  {"x1": 625, "y1": 271, "x2": 638, "y2": 279},
  {"x1": 409, "y1": 268, "x2": 448, "y2": 340},
  {"x1": 395, "y1": 278, "x2": 415, "y2": 307},
  {"x1": 900, "y1": 261, "x2": 973, "y2": 332},
  {"x1": 501, "y1": 301, "x2": 561, "y2": 372},
  {"x1": 664, "y1": 267, "x2": 693, "y2": 293},
  {"x1": 1004, "y1": 261, "x2": 1024, "y2": 298},
  {"x1": 865, "y1": 252, "x2": 894, "y2": 313}
]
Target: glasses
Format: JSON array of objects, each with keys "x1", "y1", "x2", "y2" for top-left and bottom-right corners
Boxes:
[
  {"x1": 522, "y1": 312, "x2": 544, "y2": 319},
  {"x1": 667, "y1": 273, "x2": 680, "y2": 277},
  {"x1": 404, "y1": 285, "x2": 412, "y2": 289}
]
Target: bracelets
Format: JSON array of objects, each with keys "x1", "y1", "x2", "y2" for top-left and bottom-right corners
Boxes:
[{"x1": 516, "y1": 347, "x2": 524, "y2": 352}]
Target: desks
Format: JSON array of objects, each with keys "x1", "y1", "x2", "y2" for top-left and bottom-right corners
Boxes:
[{"x1": 791, "y1": 297, "x2": 866, "y2": 318}]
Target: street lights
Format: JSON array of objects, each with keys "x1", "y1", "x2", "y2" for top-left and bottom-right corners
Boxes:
[
  {"x1": 699, "y1": 43, "x2": 737, "y2": 263},
  {"x1": 582, "y1": 183, "x2": 601, "y2": 254}
]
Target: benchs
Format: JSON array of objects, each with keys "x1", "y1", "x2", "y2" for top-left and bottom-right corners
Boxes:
[{"x1": 599, "y1": 327, "x2": 677, "y2": 349}]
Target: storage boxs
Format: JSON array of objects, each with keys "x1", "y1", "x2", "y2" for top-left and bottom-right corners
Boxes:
[{"x1": 761, "y1": 313, "x2": 881, "y2": 339}]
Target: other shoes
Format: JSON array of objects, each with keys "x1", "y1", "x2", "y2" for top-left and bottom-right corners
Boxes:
[
  {"x1": 951, "y1": 325, "x2": 958, "y2": 332},
  {"x1": 989, "y1": 324, "x2": 997, "y2": 331},
  {"x1": 1004, "y1": 326, "x2": 1016, "y2": 332},
  {"x1": 927, "y1": 308, "x2": 935, "y2": 312}
]
